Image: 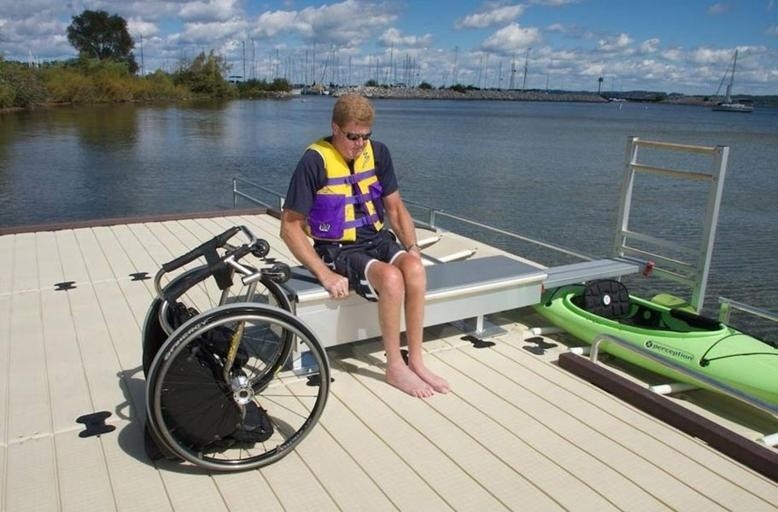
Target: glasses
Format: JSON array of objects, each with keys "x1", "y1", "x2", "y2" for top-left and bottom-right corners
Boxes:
[{"x1": 340, "y1": 128, "x2": 372, "y2": 141}]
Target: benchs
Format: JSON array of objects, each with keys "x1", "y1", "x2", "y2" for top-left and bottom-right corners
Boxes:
[{"x1": 270, "y1": 255, "x2": 549, "y2": 370}]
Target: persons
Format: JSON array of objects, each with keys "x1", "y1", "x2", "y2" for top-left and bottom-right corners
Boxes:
[{"x1": 277, "y1": 95, "x2": 458, "y2": 399}]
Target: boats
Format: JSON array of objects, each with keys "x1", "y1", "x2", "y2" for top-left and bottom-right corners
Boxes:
[
  {"x1": 535, "y1": 276, "x2": 777, "y2": 420},
  {"x1": 712, "y1": 94, "x2": 758, "y2": 112}
]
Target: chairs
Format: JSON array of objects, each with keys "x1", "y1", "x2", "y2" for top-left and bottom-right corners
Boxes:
[{"x1": 583, "y1": 279, "x2": 630, "y2": 326}]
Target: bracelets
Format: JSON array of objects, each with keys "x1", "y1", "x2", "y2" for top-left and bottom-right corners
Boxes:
[{"x1": 407, "y1": 242, "x2": 423, "y2": 254}]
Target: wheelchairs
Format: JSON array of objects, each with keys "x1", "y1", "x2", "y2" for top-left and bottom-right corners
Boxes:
[{"x1": 142, "y1": 224, "x2": 333, "y2": 471}]
